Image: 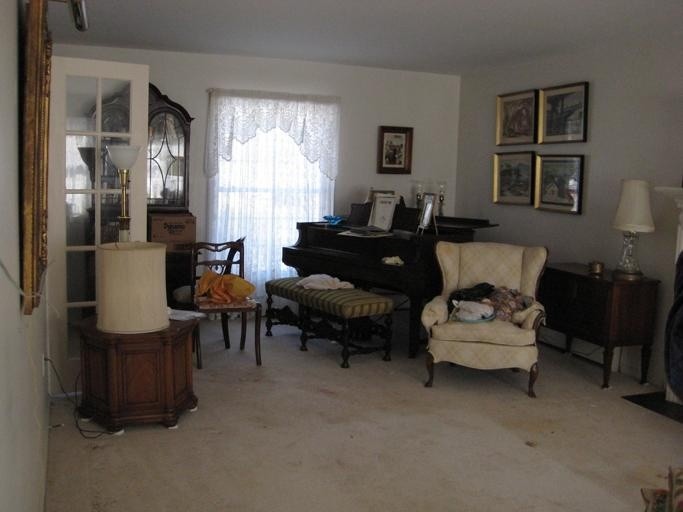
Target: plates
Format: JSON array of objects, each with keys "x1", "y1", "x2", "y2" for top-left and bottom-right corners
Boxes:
[{"x1": 322, "y1": 215, "x2": 344, "y2": 224}]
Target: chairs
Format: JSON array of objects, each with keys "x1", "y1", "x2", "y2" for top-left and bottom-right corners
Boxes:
[
  {"x1": 420, "y1": 241, "x2": 549, "y2": 402},
  {"x1": 189, "y1": 236, "x2": 264, "y2": 371}
]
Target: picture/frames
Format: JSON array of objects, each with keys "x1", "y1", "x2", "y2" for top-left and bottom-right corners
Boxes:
[
  {"x1": 374, "y1": 124, "x2": 414, "y2": 176},
  {"x1": 418, "y1": 192, "x2": 435, "y2": 230},
  {"x1": 487, "y1": 78, "x2": 589, "y2": 218}
]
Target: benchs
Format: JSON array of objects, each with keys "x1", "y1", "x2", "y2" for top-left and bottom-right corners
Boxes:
[{"x1": 266, "y1": 274, "x2": 395, "y2": 368}]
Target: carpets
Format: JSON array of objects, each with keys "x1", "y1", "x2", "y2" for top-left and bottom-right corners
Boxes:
[{"x1": 617, "y1": 386, "x2": 683, "y2": 426}]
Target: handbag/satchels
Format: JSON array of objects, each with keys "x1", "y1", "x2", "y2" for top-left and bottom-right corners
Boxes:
[{"x1": 447, "y1": 283, "x2": 494, "y2": 312}]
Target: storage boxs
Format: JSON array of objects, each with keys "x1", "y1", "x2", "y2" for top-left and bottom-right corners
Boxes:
[{"x1": 148, "y1": 210, "x2": 197, "y2": 250}]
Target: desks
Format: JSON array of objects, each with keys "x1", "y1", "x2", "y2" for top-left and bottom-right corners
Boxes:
[{"x1": 533, "y1": 259, "x2": 661, "y2": 390}]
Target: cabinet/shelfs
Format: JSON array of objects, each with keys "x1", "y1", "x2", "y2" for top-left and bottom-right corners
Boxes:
[{"x1": 84, "y1": 81, "x2": 199, "y2": 309}]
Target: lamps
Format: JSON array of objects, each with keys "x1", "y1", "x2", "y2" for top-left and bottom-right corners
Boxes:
[
  {"x1": 607, "y1": 177, "x2": 657, "y2": 283},
  {"x1": 105, "y1": 142, "x2": 142, "y2": 243}
]
